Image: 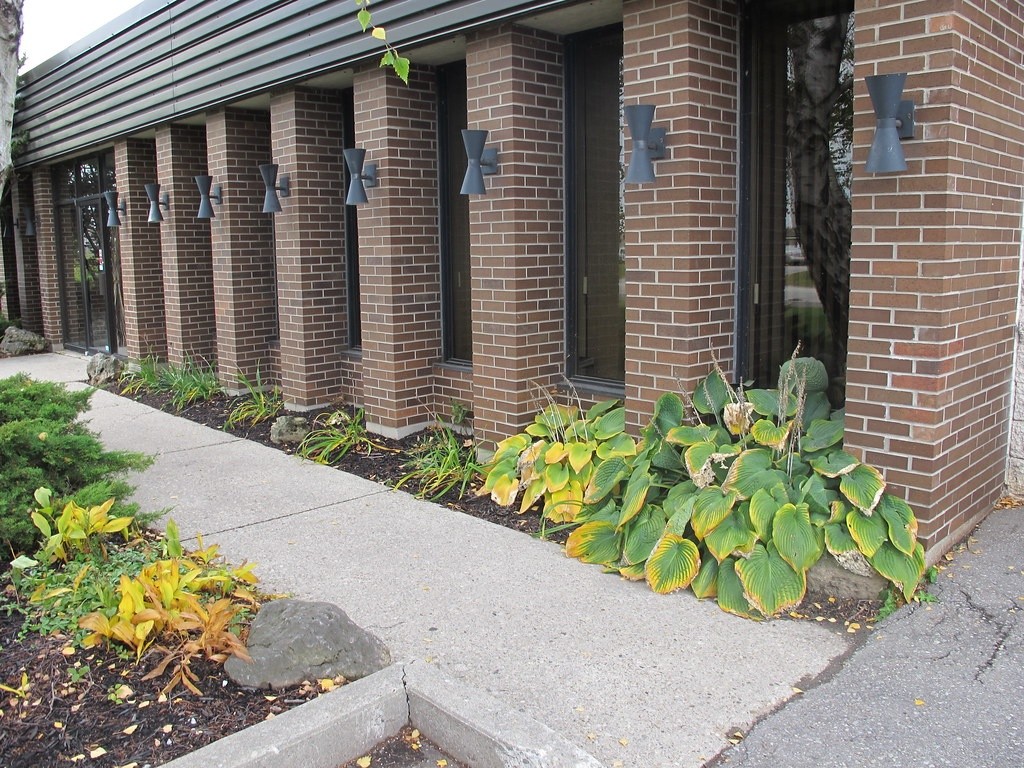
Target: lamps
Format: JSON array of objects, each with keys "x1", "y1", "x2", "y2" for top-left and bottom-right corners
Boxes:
[
  {"x1": 623, "y1": 104, "x2": 666, "y2": 184},
  {"x1": 105, "y1": 191, "x2": 126, "y2": 227},
  {"x1": 22, "y1": 206, "x2": 41, "y2": 236},
  {"x1": 259, "y1": 163, "x2": 288, "y2": 213},
  {"x1": 3, "y1": 216, "x2": 19, "y2": 238},
  {"x1": 460, "y1": 129, "x2": 497, "y2": 195},
  {"x1": 143, "y1": 184, "x2": 169, "y2": 222},
  {"x1": 864, "y1": 73, "x2": 914, "y2": 174},
  {"x1": 195, "y1": 175, "x2": 221, "y2": 218},
  {"x1": 342, "y1": 149, "x2": 376, "y2": 205}
]
